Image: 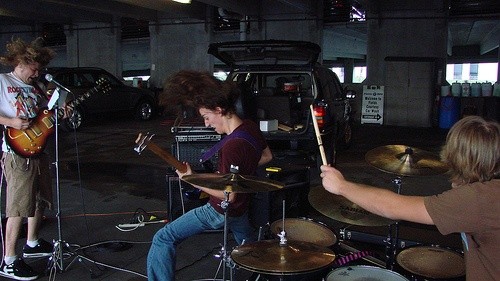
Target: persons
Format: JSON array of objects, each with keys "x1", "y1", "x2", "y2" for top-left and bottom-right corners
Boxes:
[
  {"x1": 0, "y1": 39, "x2": 73, "y2": 280},
  {"x1": 321, "y1": 115, "x2": 500, "y2": 281},
  {"x1": 147, "y1": 70, "x2": 273, "y2": 281}
]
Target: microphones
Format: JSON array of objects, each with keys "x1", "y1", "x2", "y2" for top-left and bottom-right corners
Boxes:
[{"x1": 45, "y1": 74, "x2": 71, "y2": 93}]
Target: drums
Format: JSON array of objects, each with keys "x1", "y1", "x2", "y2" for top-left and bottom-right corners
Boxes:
[
  {"x1": 269, "y1": 216, "x2": 339, "y2": 248},
  {"x1": 325, "y1": 264, "x2": 411, "y2": 281}
]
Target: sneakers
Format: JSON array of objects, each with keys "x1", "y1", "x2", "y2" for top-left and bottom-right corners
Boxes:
[
  {"x1": 0, "y1": 255, "x2": 39, "y2": 281},
  {"x1": 23, "y1": 238, "x2": 53, "y2": 258}
]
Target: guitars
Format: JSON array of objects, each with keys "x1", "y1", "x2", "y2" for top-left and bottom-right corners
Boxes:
[
  {"x1": 4, "y1": 77, "x2": 113, "y2": 160},
  {"x1": 132, "y1": 130, "x2": 219, "y2": 199}
]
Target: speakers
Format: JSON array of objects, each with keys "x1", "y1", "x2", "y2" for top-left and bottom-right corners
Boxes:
[{"x1": 165, "y1": 142, "x2": 225, "y2": 232}]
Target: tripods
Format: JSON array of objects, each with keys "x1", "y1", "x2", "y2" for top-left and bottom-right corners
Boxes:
[{"x1": 44, "y1": 86, "x2": 97, "y2": 281}]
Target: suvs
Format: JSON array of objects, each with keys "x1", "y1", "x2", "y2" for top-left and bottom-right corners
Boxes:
[
  {"x1": 207, "y1": 40, "x2": 353, "y2": 173},
  {"x1": 43, "y1": 66, "x2": 155, "y2": 130}
]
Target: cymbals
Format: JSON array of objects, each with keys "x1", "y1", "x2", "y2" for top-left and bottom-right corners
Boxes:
[
  {"x1": 230, "y1": 238, "x2": 336, "y2": 276},
  {"x1": 180, "y1": 173, "x2": 288, "y2": 193},
  {"x1": 364, "y1": 144, "x2": 452, "y2": 177},
  {"x1": 307, "y1": 166, "x2": 401, "y2": 227},
  {"x1": 395, "y1": 245, "x2": 466, "y2": 280}
]
